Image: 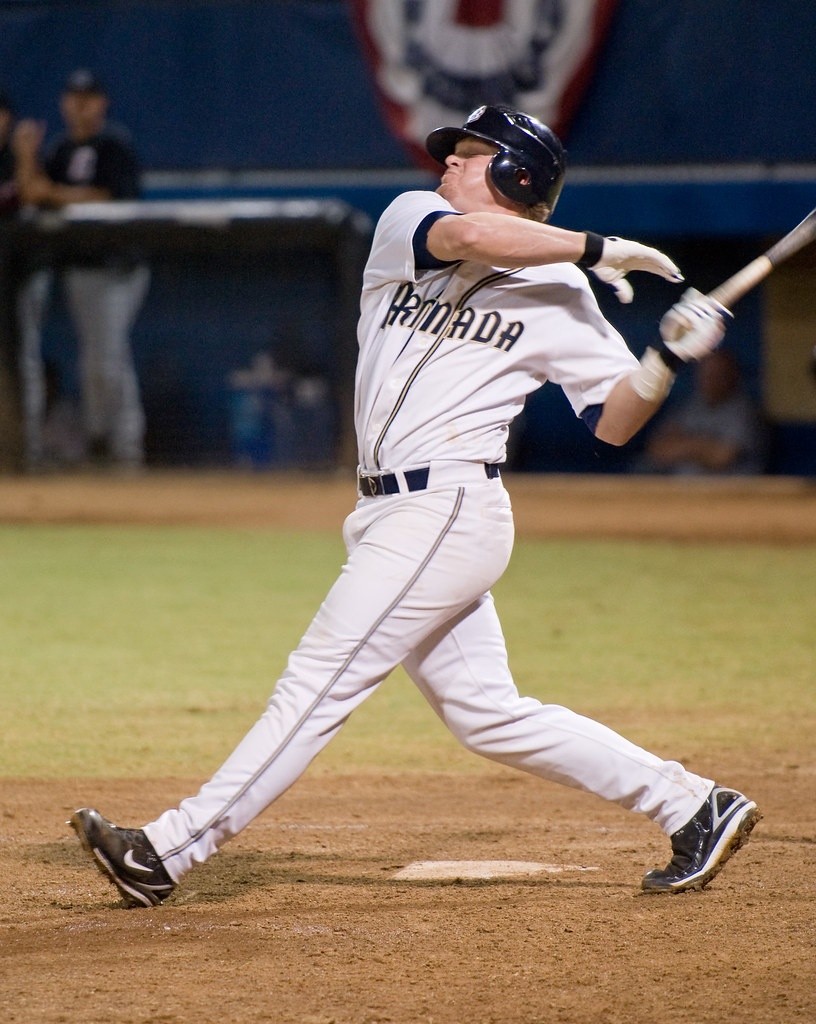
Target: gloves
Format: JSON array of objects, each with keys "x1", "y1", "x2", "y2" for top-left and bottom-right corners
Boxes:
[
  {"x1": 574, "y1": 230, "x2": 686, "y2": 303},
  {"x1": 648, "y1": 298, "x2": 728, "y2": 375}
]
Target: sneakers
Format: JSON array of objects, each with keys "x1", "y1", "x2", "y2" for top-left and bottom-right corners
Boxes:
[
  {"x1": 68, "y1": 804, "x2": 179, "y2": 908},
  {"x1": 639, "y1": 781, "x2": 765, "y2": 896}
]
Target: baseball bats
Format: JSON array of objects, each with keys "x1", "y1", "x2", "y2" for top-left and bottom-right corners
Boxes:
[{"x1": 660, "y1": 210, "x2": 816, "y2": 344}]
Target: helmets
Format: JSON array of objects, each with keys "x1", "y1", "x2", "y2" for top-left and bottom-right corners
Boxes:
[{"x1": 424, "y1": 103, "x2": 572, "y2": 223}]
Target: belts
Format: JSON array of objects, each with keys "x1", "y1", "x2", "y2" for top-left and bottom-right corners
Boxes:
[{"x1": 358, "y1": 461, "x2": 500, "y2": 497}]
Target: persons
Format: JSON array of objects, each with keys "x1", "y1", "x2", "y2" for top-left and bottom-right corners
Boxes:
[
  {"x1": 0, "y1": 82, "x2": 749, "y2": 479},
  {"x1": 70, "y1": 105, "x2": 766, "y2": 907}
]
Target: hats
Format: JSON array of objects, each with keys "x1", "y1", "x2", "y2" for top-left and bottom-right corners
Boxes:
[{"x1": 55, "y1": 69, "x2": 113, "y2": 103}]
